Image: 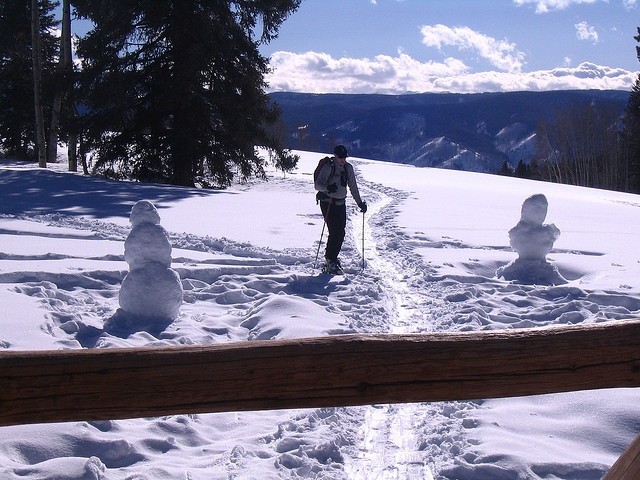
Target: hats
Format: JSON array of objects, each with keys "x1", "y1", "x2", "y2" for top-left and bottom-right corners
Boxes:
[{"x1": 334, "y1": 145, "x2": 348, "y2": 158}]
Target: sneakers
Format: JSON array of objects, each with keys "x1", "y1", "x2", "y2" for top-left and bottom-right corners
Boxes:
[{"x1": 326, "y1": 258, "x2": 343, "y2": 275}]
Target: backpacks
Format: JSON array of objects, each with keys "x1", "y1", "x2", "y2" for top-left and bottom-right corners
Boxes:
[{"x1": 314, "y1": 156, "x2": 348, "y2": 189}]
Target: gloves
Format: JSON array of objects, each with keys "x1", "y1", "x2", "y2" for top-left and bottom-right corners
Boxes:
[
  {"x1": 326, "y1": 183, "x2": 338, "y2": 193},
  {"x1": 358, "y1": 201, "x2": 367, "y2": 212}
]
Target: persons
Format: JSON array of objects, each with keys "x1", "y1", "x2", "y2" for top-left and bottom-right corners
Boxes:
[{"x1": 314, "y1": 145, "x2": 367, "y2": 273}]
